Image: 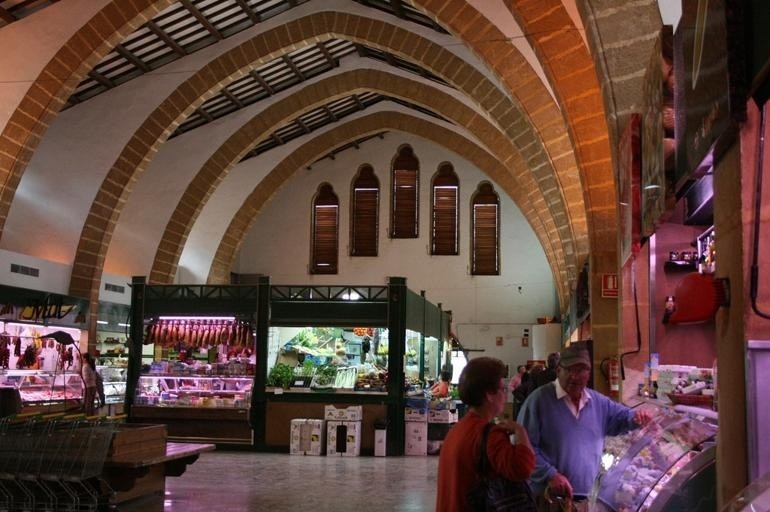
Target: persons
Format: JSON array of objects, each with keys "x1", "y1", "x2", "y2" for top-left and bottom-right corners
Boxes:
[
  {"x1": 429, "y1": 345, "x2": 650, "y2": 512},
  {"x1": 81, "y1": 353, "x2": 96, "y2": 415},
  {"x1": 179, "y1": 350, "x2": 193, "y2": 365},
  {"x1": 237, "y1": 347, "x2": 252, "y2": 363}
]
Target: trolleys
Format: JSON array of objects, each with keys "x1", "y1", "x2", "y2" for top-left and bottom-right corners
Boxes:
[{"x1": 1, "y1": 412, "x2": 126, "y2": 511}]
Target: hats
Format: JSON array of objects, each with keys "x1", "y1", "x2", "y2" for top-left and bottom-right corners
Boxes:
[{"x1": 555, "y1": 345, "x2": 592, "y2": 371}]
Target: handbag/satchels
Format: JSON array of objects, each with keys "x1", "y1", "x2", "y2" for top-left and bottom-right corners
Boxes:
[{"x1": 468, "y1": 468, "x2": 538, "y2": 512}]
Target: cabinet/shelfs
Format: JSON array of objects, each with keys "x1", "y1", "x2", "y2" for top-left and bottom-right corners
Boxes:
[
  {"x1": 594, "y1": 395, "x2": 717, "y2": 512},
  {"x1": 125, "y1": 281, "x2": 254, "y2": 448},
  {"x1": 2, "y1": 284, "x2": 125, "y2": 448}
]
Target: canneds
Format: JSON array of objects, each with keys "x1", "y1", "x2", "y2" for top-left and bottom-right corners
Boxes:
[
  {"x1": 664, "y1": 295, "x2": 675, "y2": 313},
  {"x1": 669, "y1": 250, "x2": 698, "y2": 261}
]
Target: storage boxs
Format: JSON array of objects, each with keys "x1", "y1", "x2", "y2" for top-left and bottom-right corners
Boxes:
[{"x1": 289, "y1": 403, "x2": 459, "y2": 458}]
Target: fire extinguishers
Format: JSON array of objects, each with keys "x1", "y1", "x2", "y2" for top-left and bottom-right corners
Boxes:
[{"x1": 598, "y1": 353, "x2": 620, "y2": 397}]
